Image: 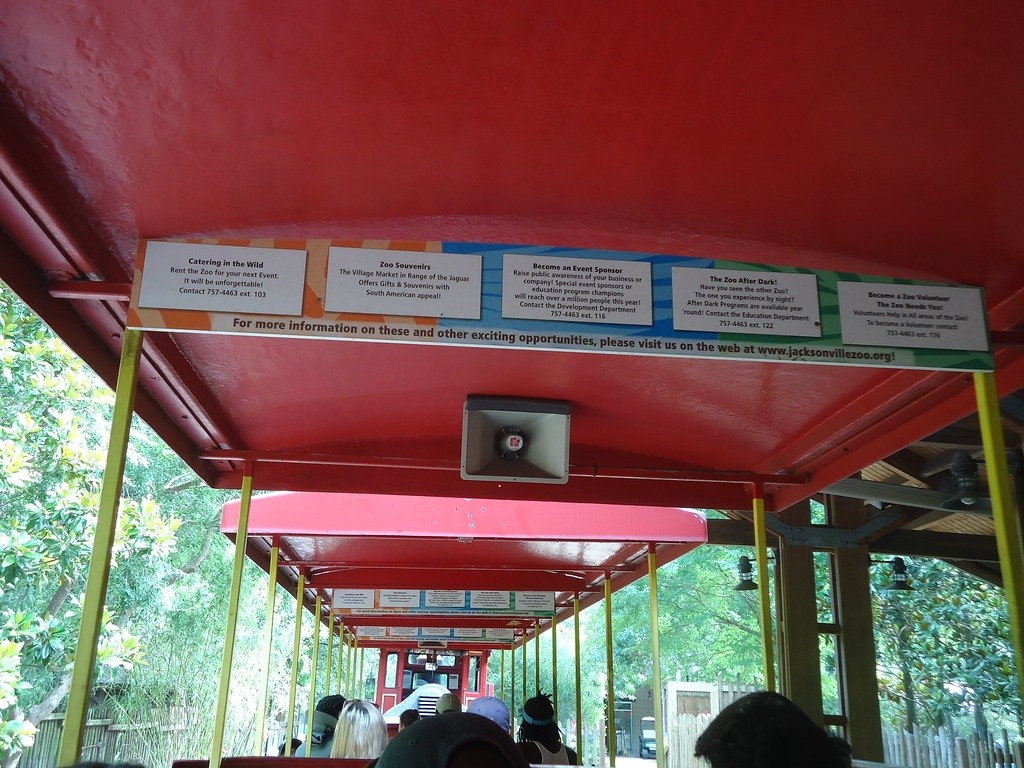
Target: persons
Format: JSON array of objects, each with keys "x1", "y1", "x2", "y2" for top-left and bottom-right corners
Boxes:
[
  {"x1": 518, "y1": 696, "x2": 577, "y2": 765},
  {"x1": 435, "y1": 693, "x2": 462, "y2": 715},
  {"x1": 696, "y1": 691, "x2": 854, "y2": 767},
  {"x1": 467, "y1": 697, "x2": 510, "y2": 731},
  {"x1": 374, "y1": 713, "x2": 531, "y2": 768},
  {"x1": 278, "y1": 695, "x2": 346, "y2": 757},
  {"x1": 331, "y1": 700, "x2": 390, "y2": 759},
  {"x1": 399, "y1": 710, "x2": 418, "y2": 732}
]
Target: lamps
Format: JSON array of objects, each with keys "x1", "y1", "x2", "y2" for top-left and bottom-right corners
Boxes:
[
  {"x1": 733, "y1": 550, "x2": 777, "y2": 591},
  {"x1": 867, "y1": 554, "x2": 915, "y2": 590},
  {"x1": 950, "y1": 447, "x2": 1024, "y2": 506}
]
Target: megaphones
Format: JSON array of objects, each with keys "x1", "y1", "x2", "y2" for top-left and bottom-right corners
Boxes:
[
  {"x1": 417, "y1": 640, "x2": 448, "y2": 648},
  {"x1": 460, "y1": 396, "x2": 572, "y2": 483}
]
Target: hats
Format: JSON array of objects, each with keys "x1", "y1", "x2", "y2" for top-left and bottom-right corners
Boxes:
[
  {"x1": 374, "y1": 712, "x2": 530, "y2": 768},
  {"x1": 436, "y1": 693, "x2": 462, "y2": 714},
  {"x1": 278, "y1": 738, "x2": 302, "y2": 755},
  {"x1": 518, "y1": 696, "x2": 554, "y2": 726},
  {"x1": 466, "y1": 696, "x2": 510, "y2": 734}
]
